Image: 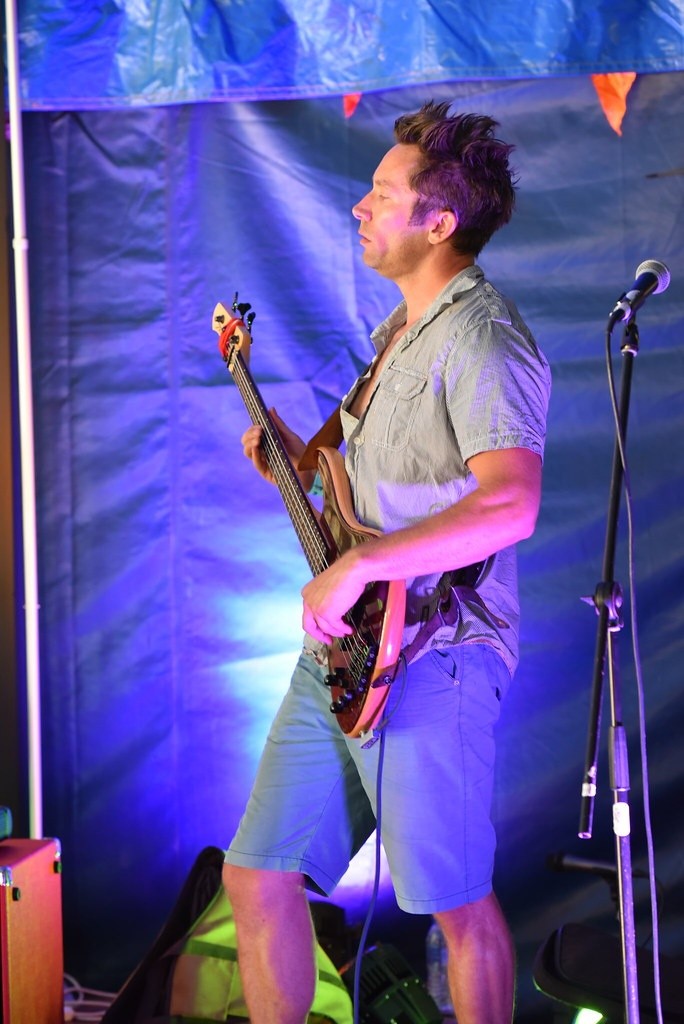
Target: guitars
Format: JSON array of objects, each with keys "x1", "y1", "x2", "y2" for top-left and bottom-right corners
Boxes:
[{"x1": 210, "y1": 296, "x2": 410, "y2": 740}]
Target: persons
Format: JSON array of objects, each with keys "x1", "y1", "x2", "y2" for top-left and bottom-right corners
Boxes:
[{"x1": 220, "y1": 98, "x2": 553, "y2": 1024}]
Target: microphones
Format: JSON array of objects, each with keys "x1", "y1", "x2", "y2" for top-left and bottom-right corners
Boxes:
[{"x1": 609, "y1": 259, "x2": 671, "y2": 321}]
